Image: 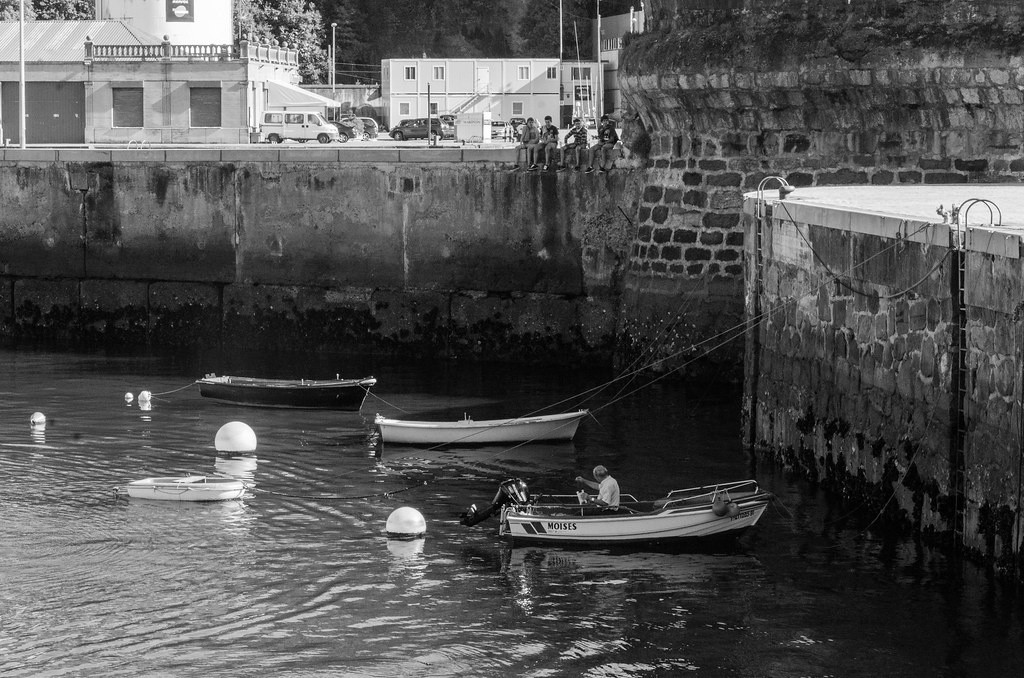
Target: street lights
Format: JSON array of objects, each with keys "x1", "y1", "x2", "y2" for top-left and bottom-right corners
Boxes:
[{"x1": 330, "y1": 22, "x2": 338, "y2": 123}]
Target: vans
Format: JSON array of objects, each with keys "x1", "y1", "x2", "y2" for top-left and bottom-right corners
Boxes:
[{"x1": 260, "y1": 110, "x2": 341, "y2": 145}]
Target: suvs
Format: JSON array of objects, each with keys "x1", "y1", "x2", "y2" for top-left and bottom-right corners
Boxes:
[
  {"x1": 510, "y1": 117, "x2": 529, "y2": 140},
  {"x1": 438, "y1": 114, "x2": 459, "y2": 139},
  {"x1": 389, "y1": 117, "x2": 450, "y2": 142}
]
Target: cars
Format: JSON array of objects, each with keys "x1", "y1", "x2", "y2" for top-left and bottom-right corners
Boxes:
[
  {"x1": 491, "y1": 120, "x2": 514, "y2": 140},
  {"x1": 292, "y1": 120, "x2": 357, "y2": 144},
  {"x1": 338, "y1": 116, "x2": 379, "y2": 142}
]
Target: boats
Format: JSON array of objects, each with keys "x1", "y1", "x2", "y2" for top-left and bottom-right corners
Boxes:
[
  {"x1": 373, "y1": 408, "x2": 592, "y2": 446},
  {"x1": 497, "y1": 537, "x2": 765, "y2": 595},
  {"x1": 124, "y1": 474, "x2": 247, "y2": 501},
  {"x1": 194, "y1": 368, "x2": 377, "y2": 412},
  {"x1": 460, "y1": 474, "x2": 774, "y2": 545}
]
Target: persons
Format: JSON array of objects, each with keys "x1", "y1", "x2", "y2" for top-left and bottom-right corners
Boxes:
[
  {"x1": 585, "y1": 115, "x2": 618, "y2": 174},
  {"x1": 511, "y1": 117, "x2": 540, "y2": 170},
  {"x1": 572, "y1": 465, "x2": 620, "y2": 515},
  {"x1": 527, "y1": 116, "x2": 559, "y2": 170},
  {"x1": 556, "y1": 119, "x2": 588, "y2": 171}
]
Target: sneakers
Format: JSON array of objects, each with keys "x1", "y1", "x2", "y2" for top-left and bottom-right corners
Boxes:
[
  {"x1": 527, "y1": 167, "x2": 529, "y2": 173},
  {"x1": 573, "y1": 165, "x2": 580, "y2": 172},
  {"x1": 584, "y1": 167, "x2": 595, "y2": 173},
  {"x1": 510, "y1": 164, "x2": 520, "y2": 171},
  {"x1": 599, "y1": 168, "x2": 605, "y2": 173},
  {"x1": 529, "y1": 164, "x2": 538, "y2": 171},
  {"x1": 556, "y1": 165, "x2": 565, "y2": 172},
  {"x1": 543, "y1": 164, "x2": 549, "y2": 172}
]
